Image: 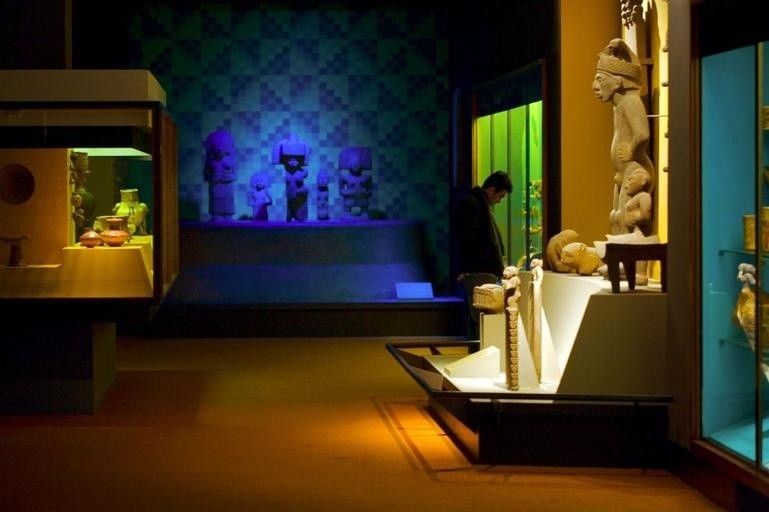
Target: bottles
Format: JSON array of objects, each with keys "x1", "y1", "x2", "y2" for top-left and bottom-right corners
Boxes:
[{"x1": 79, "y1": 189, "x2": 145, "y2": 247}]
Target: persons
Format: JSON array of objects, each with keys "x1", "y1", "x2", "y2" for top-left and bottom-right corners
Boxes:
[
  {"x1": 456, "y1": 170, "x2": 513, "y2": 349},
  {"x1": 591, "y1": 39, "x2": 656, "y2": 286},
  {"x1": 286, "y1": 158, "x2": 309, "y2": 223},
  {"x1": 337, "y1": 145, "x2": 372, "y2": 217},
  {"x1": 247, "y1": 170, "x2": 272, "y2": 220},
  {"x1": 317, "y1": 168, "x2": 329, "y2": 220},
  {"x1": 201, "y1": 128, "x2": 238, "y2": 220}
]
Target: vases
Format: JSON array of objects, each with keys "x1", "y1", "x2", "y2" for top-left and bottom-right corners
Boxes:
[{"x1": 76, "y1": 187, "x2": 149, "y2": 249}]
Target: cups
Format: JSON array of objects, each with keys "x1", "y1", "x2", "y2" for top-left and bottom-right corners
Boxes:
[{"x1": 743, "y1": 214, "x2": 755, "y2": 251}]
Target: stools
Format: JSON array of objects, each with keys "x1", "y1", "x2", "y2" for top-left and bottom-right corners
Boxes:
[{"x1": 602, "y1": 241, "x2": 668, "y2": 293}]
